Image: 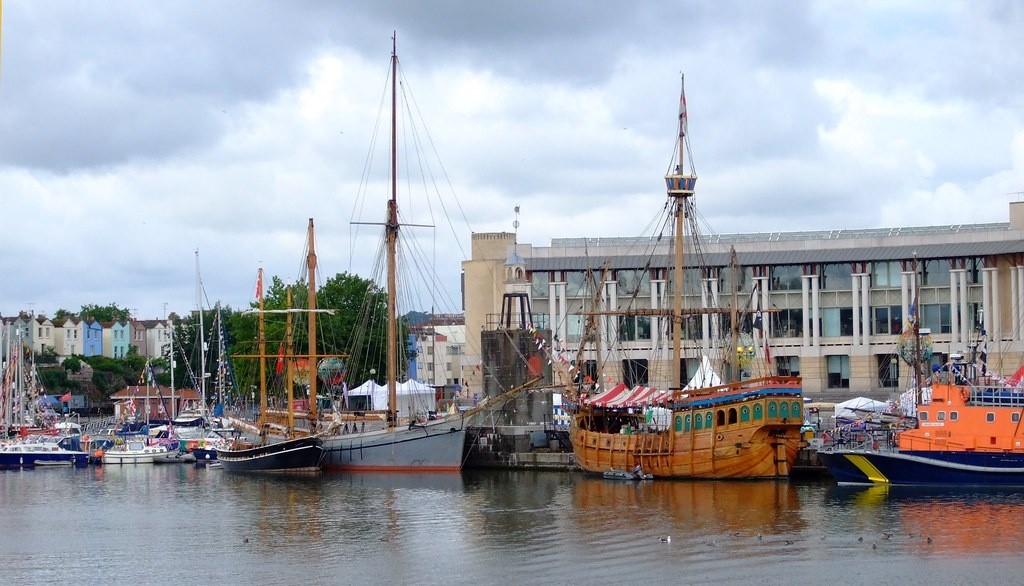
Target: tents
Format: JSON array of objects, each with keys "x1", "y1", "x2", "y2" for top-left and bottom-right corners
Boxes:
[
  {"x1": 31, "y1": 395, "x2": 58, "y2": 405},
  {"x1": 680, "y1": 353, "x2": 730, "y2": 401},
  {"x1": 581, "y1": 383, "x2": 678, "y2": 409},
  {"x1": 347, "y1": 380, "x2": 436, "y2": 418},
  {"x1": 834, "y1": 387, "x2": 931, "y2": 447}
]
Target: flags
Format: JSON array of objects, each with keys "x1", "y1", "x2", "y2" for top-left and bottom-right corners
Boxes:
[
  {"x1": 753, "y1": 301, "x2": 763, "y2": 331},
  {"x1": 765, "y1": 340, "x2": 771, "y2": 364},
  {"x1": 278, "y1": 347, "x2": 283, "y2": 373},
  {"x1": 678, "y1": 91, "x2": 688, "y2": 137},
  {"x1": 979, "y1": 342, "x2": 987, "y2": 376},
  {"x1": 907, "y1": 303, "x2": 915, "y2": 328},
  {"x1": 256, "y1": 274, "x2": 259, "y2": 297},
  {"x1": 60, "y1": 393, "x2": 70, "y2": 401}
]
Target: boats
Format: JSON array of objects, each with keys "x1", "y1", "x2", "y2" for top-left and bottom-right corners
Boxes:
[{"x1": 815, "y1": 248, "x2": 1024, "y2": 488}]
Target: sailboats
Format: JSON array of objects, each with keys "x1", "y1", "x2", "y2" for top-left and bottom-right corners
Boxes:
[
  {"x1": 563, "y1": 68, "x2": 812, "y2": 477},
  {"x1": 0, "y1": 25, "x2": 544, "y2": 470}
]
